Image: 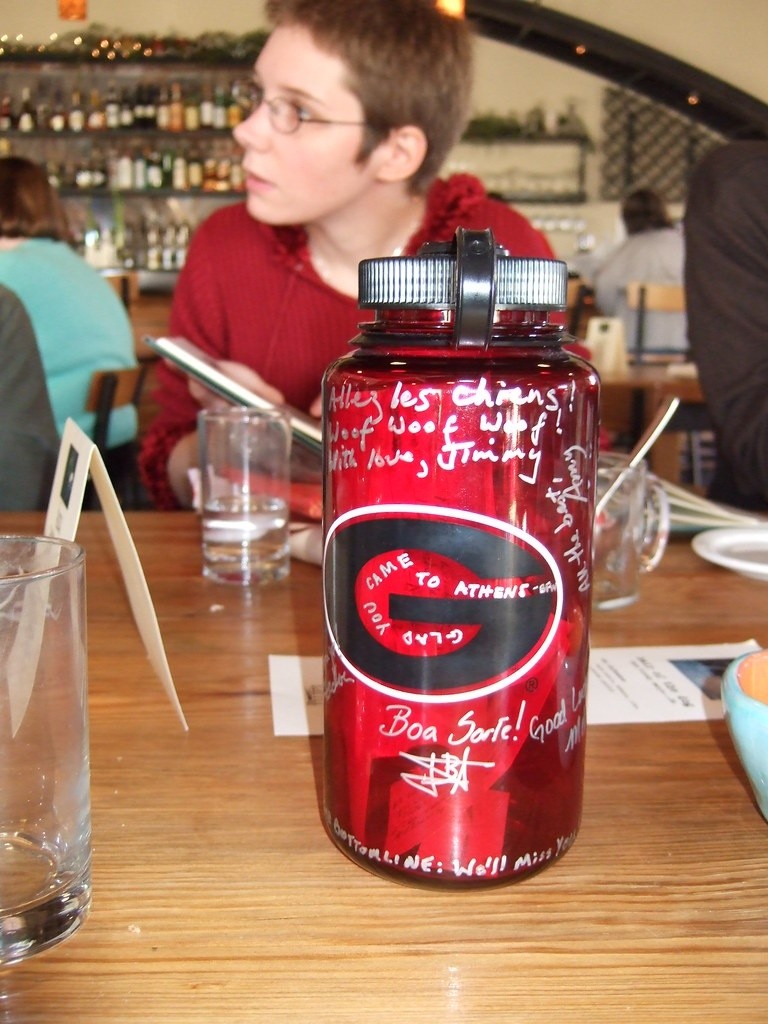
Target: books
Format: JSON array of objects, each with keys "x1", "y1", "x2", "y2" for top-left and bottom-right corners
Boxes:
[{"x1": 142, "y1": 334, "x2": 768, "y2": 555}]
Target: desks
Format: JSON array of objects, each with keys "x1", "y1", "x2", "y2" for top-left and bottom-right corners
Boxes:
[
  {"x1": 594, "y1": 367, "x2": 727, "y2": 498},
  {"x1": 0, "y1": 507, "x2": 767, "y2": 1022}
]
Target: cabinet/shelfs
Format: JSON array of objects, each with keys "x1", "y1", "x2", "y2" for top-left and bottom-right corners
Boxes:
[
  {"x1": 446, "y1": 130, "x2": 592, "y2": 208},
  {"x1": 0, "y1": 53, "x2": 263, "y2": 290}
]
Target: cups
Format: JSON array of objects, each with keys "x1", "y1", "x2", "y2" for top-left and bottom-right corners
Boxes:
[
  {"x1": 719, "y1": 650, "x2": 768, "y2": 821},
  {"x1": 196, "y1": 406, "x2": 292, "y2": 586},
  {"x1": 0, "y1": 534, "x2": 94, "y2": 966},
  {"x1": 592, "y1": 452, "x2": 670, "y2": 610}
]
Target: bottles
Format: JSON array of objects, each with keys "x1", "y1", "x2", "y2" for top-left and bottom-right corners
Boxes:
[
  {"x1": 0, "y1": 78, "x2": 257, "y2": 132},
  {"x1": 323, "y1": 226, "x2": 602, "y2": 891},
  {"x1": 84, "y1": 212, "x2": 192, "y2": 274},
  {"x1": 1, "y1": 137, "x2": 246, "y2": 193}
]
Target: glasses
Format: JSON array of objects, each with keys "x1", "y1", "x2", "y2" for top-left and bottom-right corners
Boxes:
[{"x1": 229, "y1": 78, "x2": 392, "y2": 133}]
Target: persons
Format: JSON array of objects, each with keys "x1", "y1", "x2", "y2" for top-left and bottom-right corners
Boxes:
[
  {"x1": 596, "y1": 187, "x2": 690, "y2": 362},
  {"x1": 138, "y1": 0, "x2": 594, "y2": 514},
  {"x1": 679, "y1": 138, "x2": 768, "y2": 511},
  {"x1": 0, "y1": 156, "x2": 141, "y2": 515}
]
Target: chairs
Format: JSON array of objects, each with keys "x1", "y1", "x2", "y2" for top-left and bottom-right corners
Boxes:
[{"x1": 88, "y1": 368, "x2": 146, "y2": 496}]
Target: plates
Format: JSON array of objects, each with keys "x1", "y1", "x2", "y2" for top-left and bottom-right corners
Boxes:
[{"x1": 692, "y1": 526, "x2": 768, "y2": 581}]
